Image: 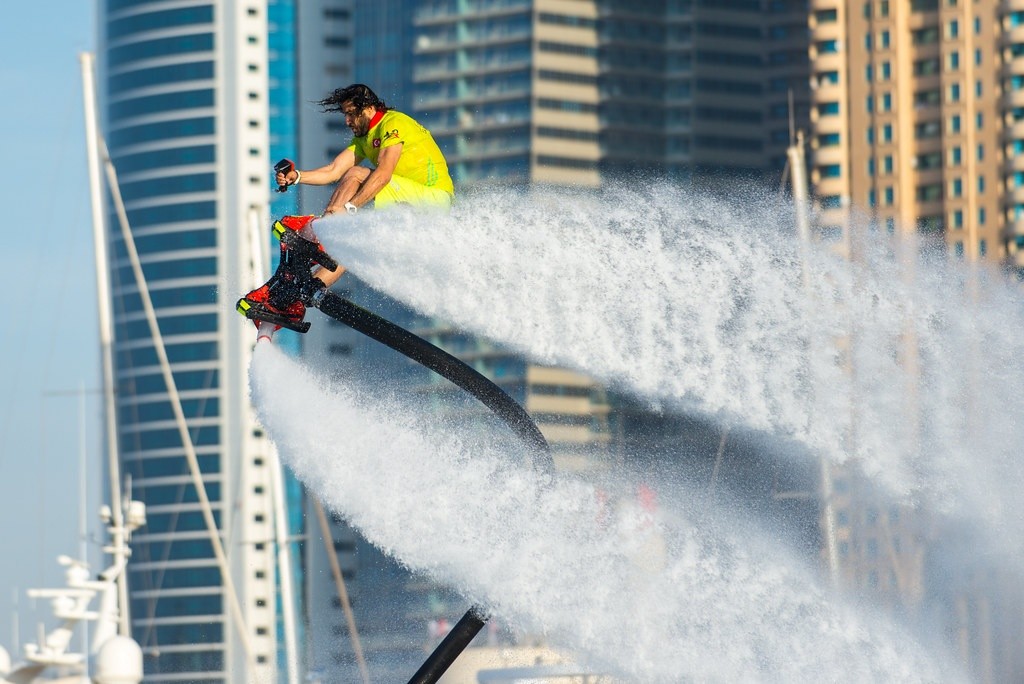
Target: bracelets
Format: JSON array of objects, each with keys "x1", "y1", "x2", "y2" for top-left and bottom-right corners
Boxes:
[{"x1": 295, "y1": 170, "x2": 300, "y2": 185}]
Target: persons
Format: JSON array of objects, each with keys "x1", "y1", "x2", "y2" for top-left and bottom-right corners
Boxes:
[{"x1": 244, "y1": 83, "x2": 452, "y2": 321}]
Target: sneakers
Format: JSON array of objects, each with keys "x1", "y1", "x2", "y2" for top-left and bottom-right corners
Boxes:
[
  {"x1": 245, "y1": 285, "x2": 306, "y2": 322},
  {"x1": 281, "y1": 215, "x2": 328, "y2": 254}
]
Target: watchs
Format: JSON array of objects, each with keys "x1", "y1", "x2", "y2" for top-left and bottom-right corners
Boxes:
[{"x1": 344, "y1": 202, "x2": 357, "y2": 215}]
